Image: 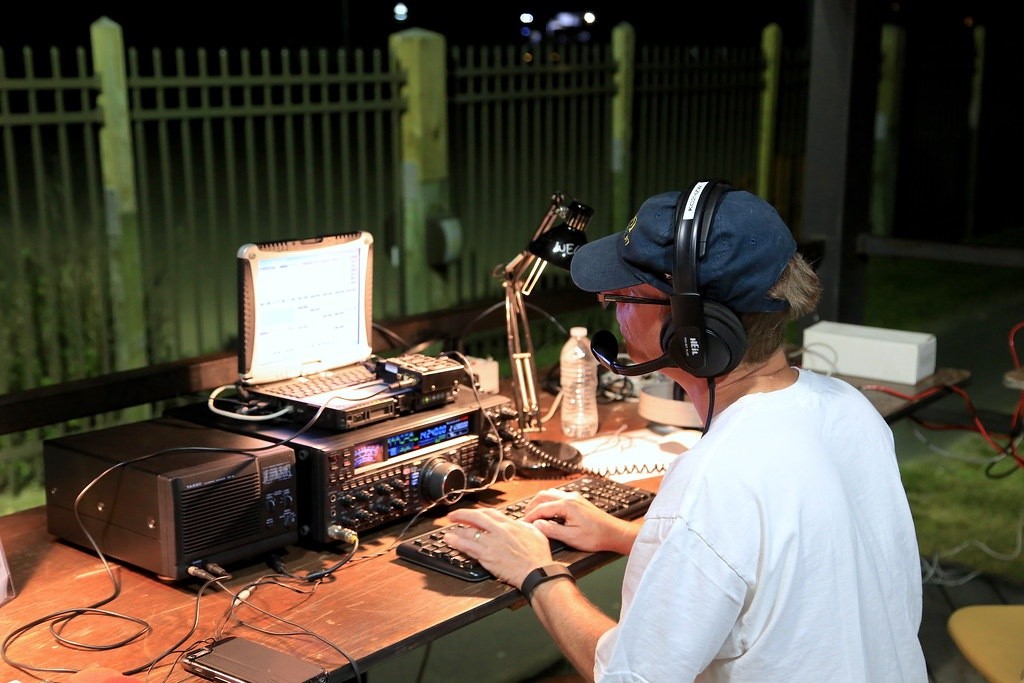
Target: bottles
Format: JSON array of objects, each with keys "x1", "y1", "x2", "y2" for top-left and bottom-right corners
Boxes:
[{"x1": 561, "y1": 327, "x2": 598, "y2": 438}]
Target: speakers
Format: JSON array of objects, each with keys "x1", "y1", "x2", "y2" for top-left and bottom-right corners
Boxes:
[{"x1": 43, "y1": 414, "x2": 298, "y2": 581}]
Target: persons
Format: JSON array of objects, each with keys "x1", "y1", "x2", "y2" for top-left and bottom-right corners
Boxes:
[{"x1": 443, "y1": 182, "x2": 929, "y2": 683}]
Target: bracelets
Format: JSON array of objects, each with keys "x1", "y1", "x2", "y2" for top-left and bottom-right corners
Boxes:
[{"x1": 520, "y1": 564, "x2": 575, "y2": 596}]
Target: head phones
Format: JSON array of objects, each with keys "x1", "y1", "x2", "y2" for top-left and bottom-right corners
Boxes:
[{"x1": 659, "y1": 179, "x2": 748, "y2": 378}]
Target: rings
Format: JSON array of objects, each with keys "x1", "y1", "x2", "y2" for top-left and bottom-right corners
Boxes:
[{"x1": 474, "y1": 531, "x2": 480, "y2": 541}]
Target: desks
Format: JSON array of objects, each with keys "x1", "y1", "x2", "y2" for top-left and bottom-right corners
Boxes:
[{"x1": 0, "y1": 370, "x2": 969, "y2": 683}]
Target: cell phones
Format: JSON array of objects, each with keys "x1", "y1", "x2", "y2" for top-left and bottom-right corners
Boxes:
[{"x1": 180, "y1": 634, "x2": 331, "y2": 683}]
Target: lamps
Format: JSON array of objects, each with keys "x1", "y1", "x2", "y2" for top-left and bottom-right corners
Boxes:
[{"x1": 492, "y1": 190, "x2": 595, "y2": 478}]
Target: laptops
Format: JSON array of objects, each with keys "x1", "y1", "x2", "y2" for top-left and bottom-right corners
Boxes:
[{"x1": 235, "y1": 229, "x2": 458, "y2": 428}]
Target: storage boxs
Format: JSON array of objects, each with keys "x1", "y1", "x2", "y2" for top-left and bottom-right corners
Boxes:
[{"x1": 802, "y1": 320, "x2": 936, "y2": 385}]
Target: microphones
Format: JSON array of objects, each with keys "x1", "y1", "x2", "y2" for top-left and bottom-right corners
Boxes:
[{"x1": 590, "y1": 329, "x2": 676, "y2": 376}]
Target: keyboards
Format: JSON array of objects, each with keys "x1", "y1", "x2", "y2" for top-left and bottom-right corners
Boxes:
[{"x1": 399, "y1": 477, "x2": 658, "y2": 582}]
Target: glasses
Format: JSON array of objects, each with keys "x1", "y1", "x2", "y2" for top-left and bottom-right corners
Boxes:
[{"x1": 597, "y1": 290, "x2": 671, "y2": 312}]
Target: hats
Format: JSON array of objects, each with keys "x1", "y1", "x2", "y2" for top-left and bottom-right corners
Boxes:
[{"x1": 570, "y1": 190, "x2": 792, "y2": 312}]
